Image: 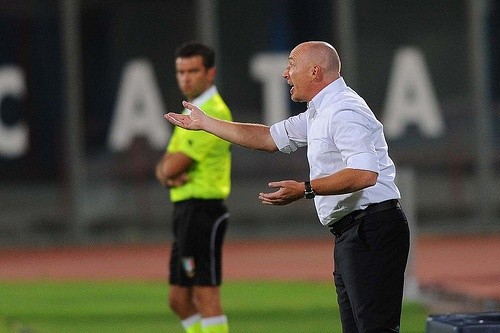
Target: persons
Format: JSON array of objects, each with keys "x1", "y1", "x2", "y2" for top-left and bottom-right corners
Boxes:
[
  {"x1": 163, "y1": 41, "x2": 411, "y2": 333},
  {"x1": 154, "y1": 41, "x2": 234, "y2": 333}
]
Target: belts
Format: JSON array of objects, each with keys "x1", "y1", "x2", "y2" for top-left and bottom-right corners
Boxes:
[{"x1": 328, "y1": 199, "x2": 400, "y2": 234}]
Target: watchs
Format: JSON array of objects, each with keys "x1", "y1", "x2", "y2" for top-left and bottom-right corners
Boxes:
[{"x1": 303, "y1": 181, "x2": 314, "y2": 200}]
